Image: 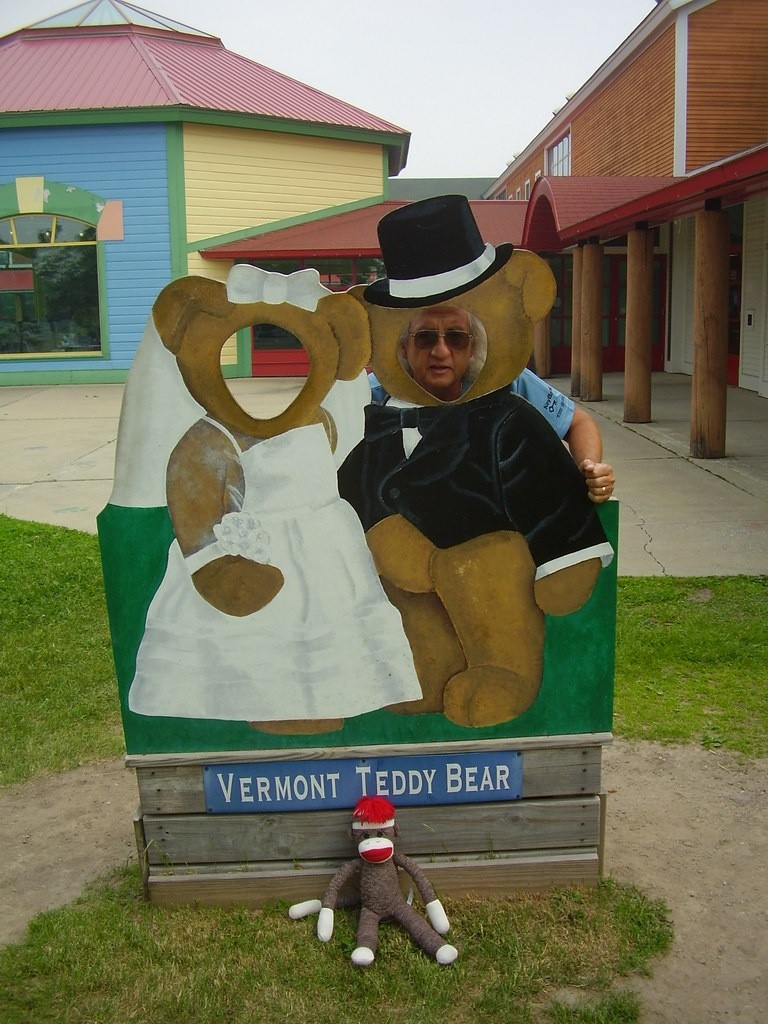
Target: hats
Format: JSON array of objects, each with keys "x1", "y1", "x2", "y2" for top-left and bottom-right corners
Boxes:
[{"x1": 362, "y1": 194, "x2": 516, "y2": 310}]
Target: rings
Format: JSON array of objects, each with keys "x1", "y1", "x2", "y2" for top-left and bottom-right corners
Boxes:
[{"x1": 601, "y1": 487, "x2": 606, "y2": 495}]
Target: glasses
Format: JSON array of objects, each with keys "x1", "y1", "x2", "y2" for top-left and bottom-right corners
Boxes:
[{"x1": 408, "y1": 329, "x2": 470, "y2": 354}]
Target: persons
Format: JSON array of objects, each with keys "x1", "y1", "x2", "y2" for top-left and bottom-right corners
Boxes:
[{"x1": 367, "y1": 305, "x2": 615, "y2": 504}]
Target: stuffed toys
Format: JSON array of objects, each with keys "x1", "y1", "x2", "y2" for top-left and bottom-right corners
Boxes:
[{"x1": 288, "y1": 795, "x2": 458, "y2": 965}]
